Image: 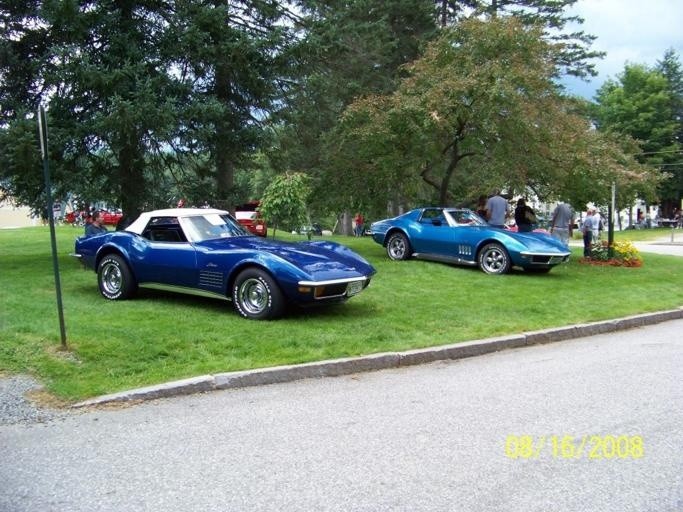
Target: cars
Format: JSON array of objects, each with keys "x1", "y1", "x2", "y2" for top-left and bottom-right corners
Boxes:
[{"x1": 233, "y1": 210, "x2": 267, "y2": 238}]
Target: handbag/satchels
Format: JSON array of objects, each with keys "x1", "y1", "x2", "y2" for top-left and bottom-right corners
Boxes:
[
  {"x1": 525, "y1": 211, "x2": 537, "y2": 224},
  {"x1": 599, "y1": 220, "x2": 604, "y2": 230}
]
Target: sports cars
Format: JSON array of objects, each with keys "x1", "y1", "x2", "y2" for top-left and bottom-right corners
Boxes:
[
  {"x1": 365, "y1": 207, "x2": 571, "y2": 275},
  {"x1": 73, "y1": 207, "x2": 377, "y2": 321}
]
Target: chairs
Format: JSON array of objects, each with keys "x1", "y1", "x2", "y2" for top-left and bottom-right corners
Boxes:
[{"x1": 150, "y1": 227, "x2": 182, "y2": 243}]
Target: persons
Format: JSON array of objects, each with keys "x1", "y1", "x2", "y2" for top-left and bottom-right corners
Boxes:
[
  {"x1": 354, "y1": 213, "x2": 362, "y2": 238},
  {"x1": 85, "y1": 211, "x2": 108, "y2": 236},
  {"x1": 476, "y1": 189, "x2": 683, "y2": 264}
]
